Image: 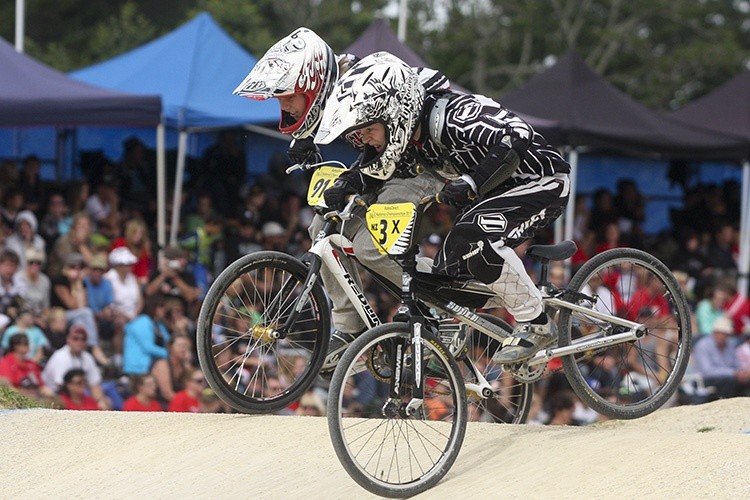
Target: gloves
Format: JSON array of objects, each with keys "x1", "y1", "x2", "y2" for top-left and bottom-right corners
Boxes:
[
  {"x1": 286, "y1": 133, "x2": 319, "y2": 166},
  {"x1": 332, "y1": 180, "x2": 359, "y2": 212},
  {"x1": 443, "y1": 175, "x2": 477, "y2": 209},
  {"x1": 362, "y1": 175, "x2": 384, "y2": 194}
]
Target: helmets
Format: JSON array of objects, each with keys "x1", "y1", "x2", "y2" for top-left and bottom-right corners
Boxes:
[
  {"x1": 313, "y1": 51, "x2": 426, "y2": 181},
  {"x1": 232, "y1": 27, "x2": 339, "y2": 141}
]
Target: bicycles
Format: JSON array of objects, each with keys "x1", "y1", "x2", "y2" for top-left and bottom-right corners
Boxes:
[
  {"x1": 326, "y1": 193, "x2": 694, "y2": 498},
  {"x1": 196, "y1": 147, "x2": 532, "y2": 427}
]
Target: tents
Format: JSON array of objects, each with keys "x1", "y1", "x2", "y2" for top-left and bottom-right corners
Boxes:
[
  {"x1": 353, "y1": 19, "x2": 561, "y2": 246},
  {"x1": 0, "y1": 38, "x2": 164, "y2": 263},
  {"x1": 492, "y1": 53, "x2": 750, "y2": 293},
  {"x1": 66, "y1": 13, "x2": 287, "y2": 246},
  {"x1": 674, "y1": 70, "x2": 750, "y2": 294}
]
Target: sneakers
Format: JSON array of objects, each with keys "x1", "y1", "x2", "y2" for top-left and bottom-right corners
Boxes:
[
  {"x1": 320, "y1": 324, "x2": 370, "y2": 372},
  {"x1": 392, "y1": 270, "x2": 415, "y2": 322},
  {"x1": 436, "y1": 307, "x2": 476, "y2": 359},
  {"x1": 493, "y1": 313, "x2": 558, "y2": 361}
]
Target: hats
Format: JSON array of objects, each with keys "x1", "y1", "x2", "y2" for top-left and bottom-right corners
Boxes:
[
  {"x1": 92, "y1": 252, "x2": 109, "y2": 271},
  {"x1": 164, "y1": 244, "x2": 184, "y2": 257},
  {"x1": 262, "y1": 222, "x2": 285, "y2": 236},
  {"x1": 27, "y1": 246, "x2": 46, "y2": 263},
  {"x1": 68, "y1": 325, "x2": 86, "y2": 337},
  {"x1": 64, "y1": 254, "x2": 85, "y2": 267},
  {"x1": 108, "y1": 246, "x2": 136, "y2": 265}
]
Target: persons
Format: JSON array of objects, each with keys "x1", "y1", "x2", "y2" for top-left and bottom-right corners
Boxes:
[
  {"x1": 233, "y1": 27, "x2": 384, "y2": 381},
  {"x1": 314, "y1": 51, "x2": 570, "y2": 362},
  {"x1": 0, "y1": 136, "x2": 750, "y2": 425}
]
